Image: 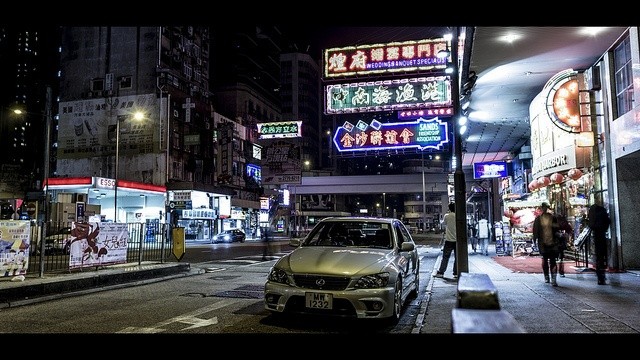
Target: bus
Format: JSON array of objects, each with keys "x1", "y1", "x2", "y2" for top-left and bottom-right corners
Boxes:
[{"x1": 289, "y1": 210, "x2": 351, "y2": 238}]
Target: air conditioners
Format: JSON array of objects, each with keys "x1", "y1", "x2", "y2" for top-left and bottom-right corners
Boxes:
[
  {"x1": 118, "y1": 74, "x2": 133, "y2": 90},
  {"x1": 90, "y1": 77, "x2": 105, "y2": 92}
]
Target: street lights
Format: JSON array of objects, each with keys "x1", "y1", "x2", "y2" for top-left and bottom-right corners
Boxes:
[
  {"x1": 295, "y1": 161, "x2": 310, "y2": 210},
  {"x1": 14, "y1": 109, "x2": 50, "y2": 221},
  {"x1": 115, "y1": 111, "x2": 144, "y2": 222}
]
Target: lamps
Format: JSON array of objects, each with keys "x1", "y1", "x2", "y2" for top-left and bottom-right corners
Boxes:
[{"x1": 578, "y1": 131, "x2": 603, "y2": 147}]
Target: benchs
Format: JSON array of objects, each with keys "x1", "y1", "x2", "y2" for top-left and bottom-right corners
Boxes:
[
  {"x1": 458, "y1": 272, "x2": 500, "y2": 310},
  {"x1": 452, "y1": 308, "x2": 526, "y2": 334}
]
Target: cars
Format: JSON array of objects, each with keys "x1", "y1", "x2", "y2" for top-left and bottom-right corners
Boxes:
[
  {"x1": 211, "y1": 229, "x2": 246, "y2": 243},
  {"x1": 19, "y1": 212, "x2": 28, "y2": 219},
  {"x1": 264, "y1": 216, "x2": 420, "y2": 323}
]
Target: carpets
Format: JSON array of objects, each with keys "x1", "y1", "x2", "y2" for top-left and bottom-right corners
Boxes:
[{"x1": 491, "y1": 254, "x2": 618, "y2": 273}]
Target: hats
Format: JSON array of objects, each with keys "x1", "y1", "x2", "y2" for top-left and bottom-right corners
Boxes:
[{"x1": 541, "y1": 203, "x2": 550, "y2": 208}]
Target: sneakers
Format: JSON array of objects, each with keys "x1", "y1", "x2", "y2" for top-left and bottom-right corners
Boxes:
[
  {"x1": 433, "y1": 271, "x2": 443, "y2": 278},
  {"x1": 560, "y1": 274, "x2": 565, "y2": 277},
  {"x1": 552, "y1": 278, "x2": 557, "y2": 286},
  {"x1": 453, "y1": 275, "x2": 457, "y2": 277},
  {"x1": 545, "y1": 276, "x2": 549, "y2": 283}
]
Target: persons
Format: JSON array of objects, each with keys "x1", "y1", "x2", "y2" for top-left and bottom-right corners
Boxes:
[
  {"x1": 261, "y1": 221, "x2": 277, "y2": 261},
  {"x1": 475, "y1": 213, "x2": 493, "y2": 256},
  {"x1": 585, "y1": 191, "x2": 626, "y2": 288},
  {"x1": 469, "y1": 219, "x2": 480, "y2": 251},
  {"x1": 553, "y1": 213, "x2": 571, "y2": 277},
  {"x1": 432, "y1": 202, "x2": 457, "y2": 278},
  {"x1": 532, "y1": 202, "x2": 564, "y2": 287}
]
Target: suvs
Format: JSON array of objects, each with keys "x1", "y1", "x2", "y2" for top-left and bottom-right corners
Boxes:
[{"x1": 38, "y1": 227, "x2": 71, "y2": 254}]
